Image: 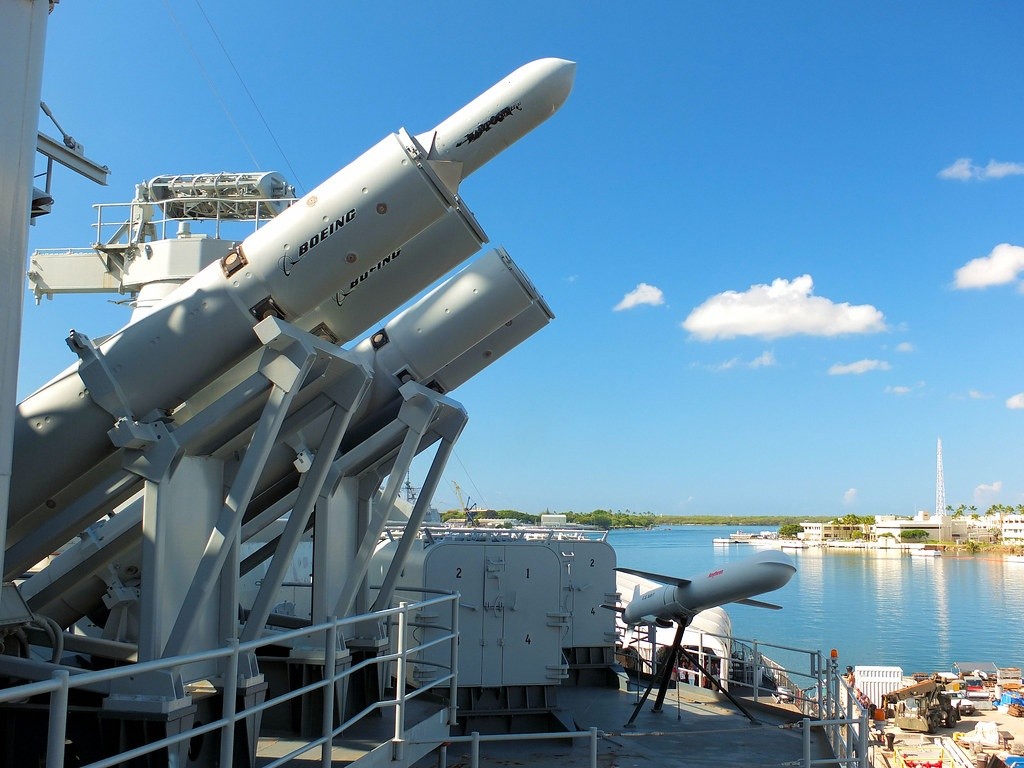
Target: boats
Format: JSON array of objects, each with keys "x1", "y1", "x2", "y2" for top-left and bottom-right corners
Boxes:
[{"x1": 909, "y1": 549, "x2": 942, "y2": 556}]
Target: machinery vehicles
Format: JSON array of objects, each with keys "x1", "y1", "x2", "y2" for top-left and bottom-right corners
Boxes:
[{"x1": 873, "y1": 670, "x2": 962, "y2": 734}]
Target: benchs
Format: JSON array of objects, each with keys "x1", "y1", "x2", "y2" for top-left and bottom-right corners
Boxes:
[{"x1": 997, "y1": 731, "x2": 1014, "y2": 751}]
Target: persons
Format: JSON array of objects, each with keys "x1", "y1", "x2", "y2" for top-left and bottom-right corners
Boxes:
[
  {"x1": 680, "y1": 662, "x2": 719, "y2": 685},
  {"x1": 848, "y1": 672, "x2": 870, "y2": 709},
  {"x1": 732, "y1": 651, "x2": 742, "y2": 687}
]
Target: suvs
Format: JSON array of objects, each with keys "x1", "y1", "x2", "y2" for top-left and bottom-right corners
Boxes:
[{"x1": 940, "y1": 692, "x2": 976, "y2": 718}]
